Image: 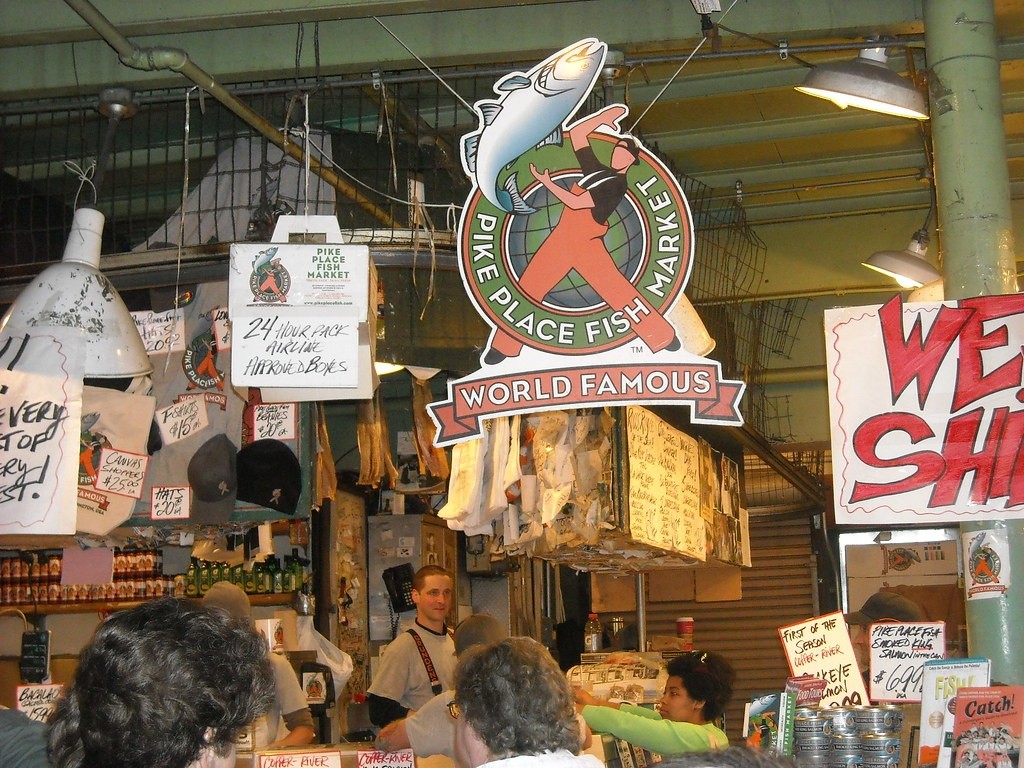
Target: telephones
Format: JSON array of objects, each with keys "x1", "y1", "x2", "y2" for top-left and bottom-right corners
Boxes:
[{"x1": 382, "y1": 563, "x2": 419, "y2": 612}]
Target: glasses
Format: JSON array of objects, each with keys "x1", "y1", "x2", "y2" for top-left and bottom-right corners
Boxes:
[
  {"x1": 447, "y1": 700, "x2": 460, "y2": 719},
  {"x1": 685, "y1": 649, "x2": 713, "y2": 674}
]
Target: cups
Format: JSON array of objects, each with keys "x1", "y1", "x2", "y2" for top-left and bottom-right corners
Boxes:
[{"x1": 675, "y1": 616, "x2": 694, "y2": 651}]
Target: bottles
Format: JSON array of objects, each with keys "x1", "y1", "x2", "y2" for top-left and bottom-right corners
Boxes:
[
  {"x1": 0, "y1": 543, "x2": 303, "y2": 601},
  {"x1": 584, "y1": 614, "x2": 603, "y2": 653}
]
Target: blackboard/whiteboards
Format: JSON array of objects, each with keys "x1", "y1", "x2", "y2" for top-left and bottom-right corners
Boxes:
[{"x1": 230, "y1": 318, "x2": 359, "y2": 389}]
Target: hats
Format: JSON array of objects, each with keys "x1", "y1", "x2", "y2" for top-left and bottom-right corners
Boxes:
[
  {"x1": 843, "y1": 591, "x2": 921, "y2": 628},
  {"x1": 201, "y1": 580, "x2": 251, "y2": 618},
  {"x1": 452, "y1": 614, "x2": 509, "y2": 656},
  {"x1": 235, "y1": 438, "x2": 302, "y2": 516},
  {"x1": 186, "y1": 433, "x2": 239, "y2": 503}
]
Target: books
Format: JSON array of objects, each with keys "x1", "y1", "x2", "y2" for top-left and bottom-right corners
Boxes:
[{"x1": 918, "y1": 658, "x2": 1024, "y2": 768}]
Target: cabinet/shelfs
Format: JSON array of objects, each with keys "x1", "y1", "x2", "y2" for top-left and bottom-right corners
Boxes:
[{"x1": 367, "y1": 513, "x2": 460, "y2": 640}]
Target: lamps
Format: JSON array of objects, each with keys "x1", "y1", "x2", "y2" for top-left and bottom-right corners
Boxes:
[
  {"x1": 861, "y1": 230, "x2": 945, "y2": 288},
  {"x1": 793, "y1": 34, "x2": 934, "y2": 120},
  {"x1": 0, "y1": 208, "x2": 153, "y2": 378}
]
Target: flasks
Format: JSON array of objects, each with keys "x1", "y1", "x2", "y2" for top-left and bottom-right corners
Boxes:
[{"x1": 609, "y1": 616, "x2": 623, "y2": 641}]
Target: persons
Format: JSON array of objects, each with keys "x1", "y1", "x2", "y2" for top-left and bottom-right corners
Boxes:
[
  {"x1": 844, "y1": 592, "x2": 923, "y2": 705},
  {"x1": 366, "y1": 565, "x2": 456, "y2": 730},
  {"x1": 646, "y1": 746, "x2": 806, "y2": 768},
  {"x1": 447, "y1": 637, "x2": 605, "y2": 768},
  {"x1": 575, "y1": 649, "x2": 737, "y2": 754},
  {"x1": 0, "y1": 597, "x2": 274, "y2": 768},
  {"x1": 201, "y1": 581, "x2": 315, "y2": 751},
  {"x1": 376, "y1": 613, "x2": 593, "y2": 762}
]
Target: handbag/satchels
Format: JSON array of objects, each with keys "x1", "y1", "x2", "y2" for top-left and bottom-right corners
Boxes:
[
  {"x1": 301, "y1": 660, "x2": 334, "y2": 718},
  {"x1": 298, "y1": 615, "x2": 354, "y2": 703},
  {"x1": 75, "y1": 376, "x2": 155, "y2": 537}
]
